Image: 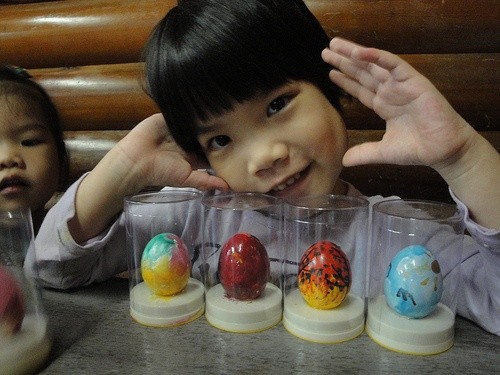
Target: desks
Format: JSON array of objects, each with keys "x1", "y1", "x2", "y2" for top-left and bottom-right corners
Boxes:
[{"x1": 0, "y1": 266, "x2": 500, "y2": 375}]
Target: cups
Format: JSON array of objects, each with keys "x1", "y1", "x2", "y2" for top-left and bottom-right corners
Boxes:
[
  {"x1": 283, "y1": 195, "x2": 369, "y2": 342},
  {"x1": 202, "y1": 193, "x2": 282, "y2": 332},
  {"x1": 125, "y1": 191, "x2": 204, "y2": 326},
  {"x1": 0, "y1": 209, "x2": 52, "y2": 375},
  {"x1": 367, "y1": 200, "x2": 465, "y2": 354}
]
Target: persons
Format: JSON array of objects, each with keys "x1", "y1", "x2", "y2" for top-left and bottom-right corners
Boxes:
[
  {"x1": 0, "y1": 64, "x2": 71, "y2": 231},
  {"x1": 24, "y1": 0, "x2": 500, "y2": 341}
]
